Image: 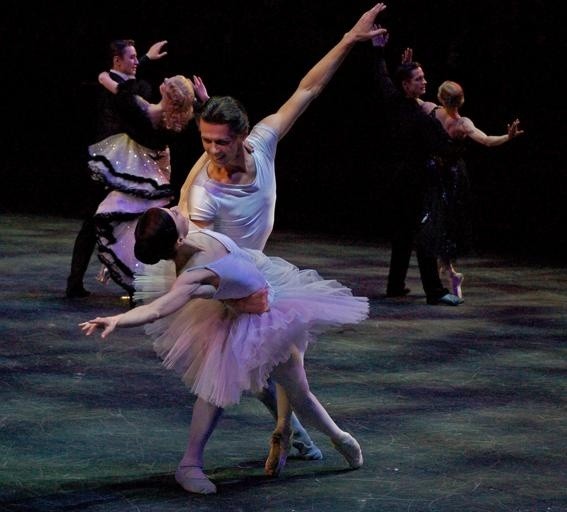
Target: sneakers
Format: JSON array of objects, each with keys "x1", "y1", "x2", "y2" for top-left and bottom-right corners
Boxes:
[
  {"x1": 265, "y1": 428, "x2": 290, "y2": 477},
  {"x1": 66, "y1": 285, "x2": 91, "y2": 298},
  {"x1": 387, "y1": 288, "x2": 410, "y2": 298},
  {"x1": 453, "y1": 272, "x2": 464, "y2": 300},
  {"x1": 427, "y1": 293, "x2": 464, "y2": 306},
  {"x1": 335, "y1": 432, "x2": 363, "y2": 469},
  {"x1": 175, "y1": 461, "x2": 217, "y2": 495},
  {"x1": 292, "y1": 433, "x2": 323, "y2": 460}
]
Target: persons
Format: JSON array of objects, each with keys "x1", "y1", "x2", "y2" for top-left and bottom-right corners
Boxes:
[
  {"x1": 367, "y1": 22, "x2": 468, "y2": 308},
  {"x1": 85, "y1": 66, "x2": 212, "y2": 307},
  {"x1": 77, "y1": 206, "x2": 374, "y2": 481},
  {"x1": 168, "y1": 2, "x2": 393, "y2": 495},
  {"x1": 63, "y1": 36, "x2": 171, "y2": 300},
  {"x1": 398, "y1": 43, "x2": 527, "y2": 302}
]
